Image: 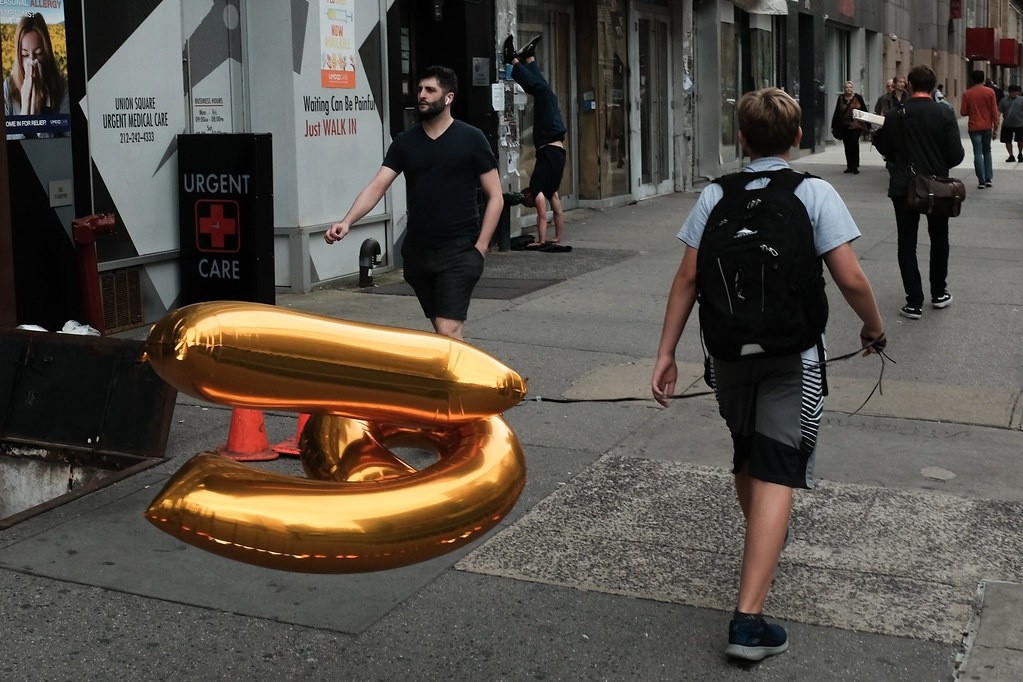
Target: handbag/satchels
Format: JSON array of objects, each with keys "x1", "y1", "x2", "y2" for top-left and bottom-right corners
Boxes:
[
  {"x1": 832, "y1": 123, "x2": 844, "y2": 140},
  {"x1": 905, "y1": 168, "x2": 967, "y2": 216}
]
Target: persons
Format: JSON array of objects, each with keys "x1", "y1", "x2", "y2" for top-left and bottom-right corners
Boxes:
[
  {"x1": 503, "y1": 34, "x2": 567, "y2": 249},
  {"x1": 3, "y1": 13, "x2": 72, "y2": 117},
  {"x1": 650, "y1": 88, "x2": 888, "y2": 660},
  {"x1": 324, "y1": 67, "x2": 504, "y2": 345},
  {"x1": 831, "y1": 65, "x2": 1023, "y2": 319}
]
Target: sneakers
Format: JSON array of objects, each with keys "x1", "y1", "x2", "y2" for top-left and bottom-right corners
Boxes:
[
  {"x1": 784, "y1": 528, "x2": 790, "y2": 546},
  {"x1": 725, "y1": 614, "x2": 788, "y2": 660},
  {"x1": 518, "y1": 34, "x2": 541, "y2": 59},
  {"x1": 978, "y1": 183, "x2": 985, "y2": 188},
  {"x1": 899, "y1": 303, "x2": 922, "y2": 319},
  {"x1": 985, "y1": 179, "x2": 992, "y2": 185},
  {"x1": 931, "y1": 290, "x2": 953, "y2": 308},
  {"x1": 503, "y1": 35, "x2": 518, "y2": 64}
]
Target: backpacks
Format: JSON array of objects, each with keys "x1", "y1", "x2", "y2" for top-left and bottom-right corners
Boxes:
[{"x1": 694, "y1": 168, "x2": 831, "y2": 397}]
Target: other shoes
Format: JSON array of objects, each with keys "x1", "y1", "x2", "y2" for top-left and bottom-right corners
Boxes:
[
  {"x1": 853, "y1": 168, "x2": 859, "y2": 174},
  {"x1": 1018, "y1": 156, "x2": 1023, "y2": 162},
  {"x1": 844, "y1": 168, "x2": 853, "y2": 172},
  {"x1": 1006, "y1": 157, "x2": 1015, "y2": 162}
]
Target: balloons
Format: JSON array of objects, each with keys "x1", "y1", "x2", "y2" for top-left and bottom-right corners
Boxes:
[{"x1": 138, "y1": 299, "x2": 528, "y2": 575}]
